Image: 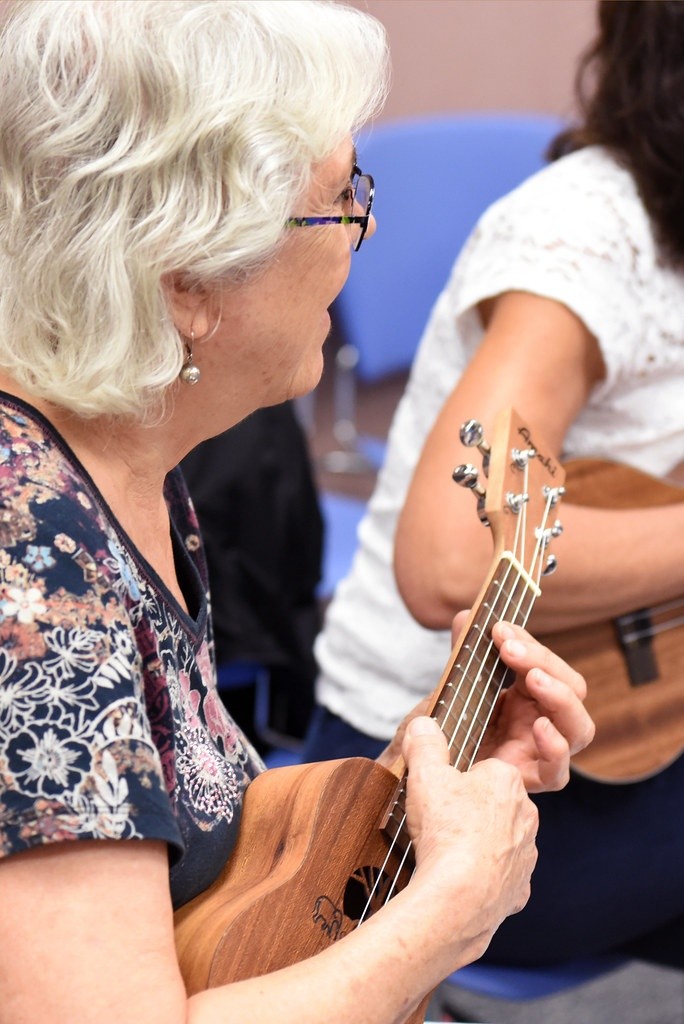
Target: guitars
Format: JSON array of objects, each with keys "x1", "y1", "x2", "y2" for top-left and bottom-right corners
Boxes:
[
  {"x1": 171, "y1": 407, "x2": 570, "y2": 1023},
  {"x1": 534, "y1": 456, "x2": 684, "y2": 785}
]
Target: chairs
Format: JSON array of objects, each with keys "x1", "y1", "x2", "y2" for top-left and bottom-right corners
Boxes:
[{"x1": 217, "y1": 111, "x2": 636, "y2": 1021}]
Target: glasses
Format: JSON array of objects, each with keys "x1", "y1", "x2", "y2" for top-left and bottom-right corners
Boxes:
[{"x1": 286, "y1": 164, "x2": 376, "y2": 252}]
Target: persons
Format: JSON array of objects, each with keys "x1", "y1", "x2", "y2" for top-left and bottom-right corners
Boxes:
[
  {"x1": 0, "y1": 0, "x2": 597, "y2": 1023},
  {"x1": 304, "y1": 0, "x2": 684, "y2": 976}
]
